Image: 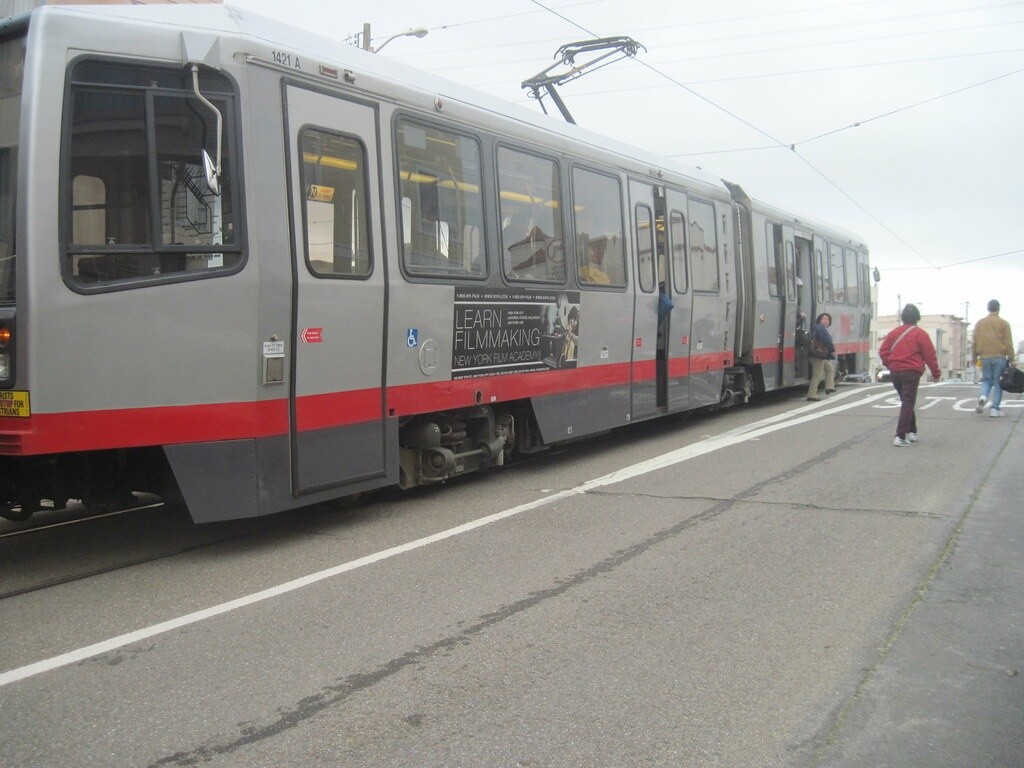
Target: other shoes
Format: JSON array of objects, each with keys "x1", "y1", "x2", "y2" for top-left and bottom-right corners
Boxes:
[
  {"x1": 807, "y1": 397, "x2": 822, "y2": 401},
  {"x1": 825, "y1": 389, "x2": 836, "y2": 394},
  {"x1": 892, "y1": 435, "x2": 912, "y2": 447},
  {"x1": 909, "y1": 432, "x2": 918, "y2": 441}
]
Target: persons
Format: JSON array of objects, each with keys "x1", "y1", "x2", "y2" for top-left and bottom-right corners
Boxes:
[
  {"x1": 972, "y1": 298, "x2": 1016, "y2": 418},
  {"x1": 879, "y1": 304, "x2": 942, "y2": 446},
  {"x1": 805, "y1": 313, "x2": 837, "y2": 401}
]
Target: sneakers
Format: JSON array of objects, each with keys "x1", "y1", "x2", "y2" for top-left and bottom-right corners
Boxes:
[
  {"x1": 990, "y1": 408, "x2": 1005, "y2": 418},
  {"x1": 975, "y1": 395, "x2": 987, "y2": 415}
]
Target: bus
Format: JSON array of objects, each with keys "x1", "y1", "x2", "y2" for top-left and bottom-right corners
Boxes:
[{"x1": 3, "y1": 1, "x2": 880, "y2": 524}]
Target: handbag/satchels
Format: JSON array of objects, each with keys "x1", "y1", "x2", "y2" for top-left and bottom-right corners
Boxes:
[
  {"x1": 810, "y1": 337, "x2": 830, "y2": 358},
  {"x1": 1000, "y1": 360, "x2": 1024, "y2": 394}
]
